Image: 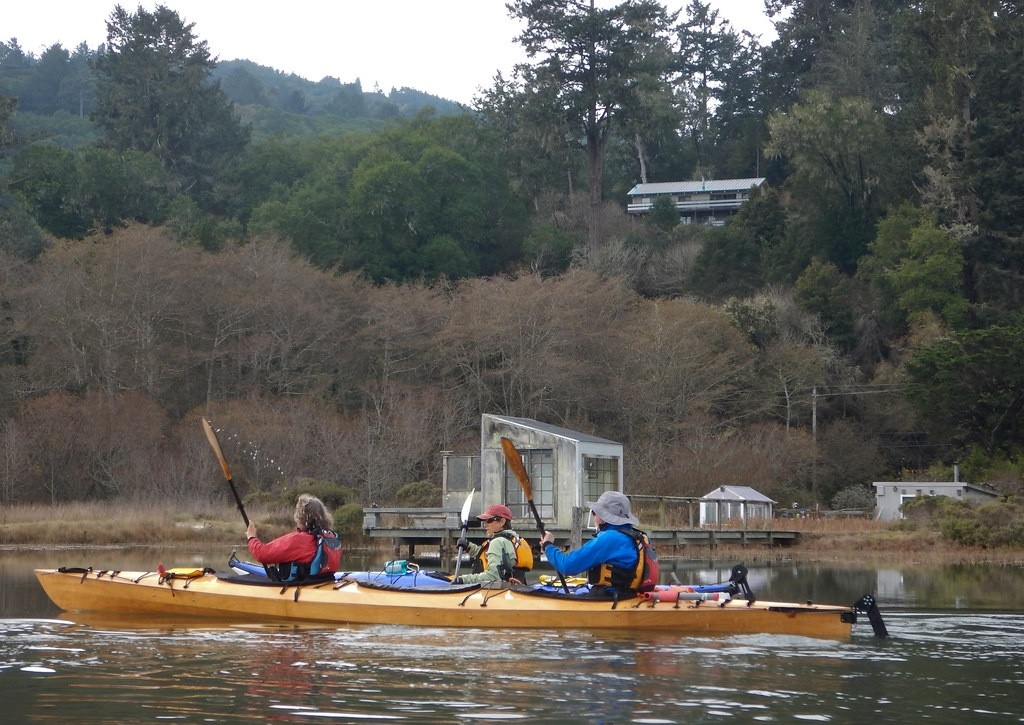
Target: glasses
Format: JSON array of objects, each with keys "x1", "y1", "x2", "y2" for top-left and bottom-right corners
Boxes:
[
  {"x1": 487, "y1": 517, "x2": 501, "y2": 523},
  {"x1": 591, "y1": 510, "x2": 596, "y2": 515}
]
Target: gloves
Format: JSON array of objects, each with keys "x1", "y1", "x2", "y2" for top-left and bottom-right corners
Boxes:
[
  {"x1": 451, "y1": 576, "x2": 463, "y2": 584},
  {"x1": 456, "y1": 537, "x2": 469, "y2": 551}
]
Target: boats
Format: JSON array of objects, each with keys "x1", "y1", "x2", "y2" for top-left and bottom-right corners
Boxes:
[{"x1": 35, "y1": 566, "x2": 855, "y2": 640}]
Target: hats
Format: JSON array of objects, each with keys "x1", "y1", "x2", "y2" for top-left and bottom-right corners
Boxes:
[
  {"x1": 476, "y1": 504, "x2": 512, "y2": 521},
  {"x1": 586, "y1": 491, "x2": 639, "y2": 525}
]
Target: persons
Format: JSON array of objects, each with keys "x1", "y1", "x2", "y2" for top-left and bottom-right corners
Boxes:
[
  {"x1": 246, "y1": 493, "x2": 342, "y2": 582},
  {"x1": 539, "y1": 490, "x2": 659, "y2": 600},
  {"x1": 451, "y1": 503, "x2": 533, "y2": 585}
]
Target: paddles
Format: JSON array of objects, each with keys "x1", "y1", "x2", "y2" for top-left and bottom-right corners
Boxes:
[
  {"x1": 452, "y1": 487, "x2": 475, "y2": 583},
  {"x1": 499, "y1": 436, "x2": 572, "y2": 597},
  {"x1": 201, "y1": 418, "x2": 271, "y2": 579}
]
